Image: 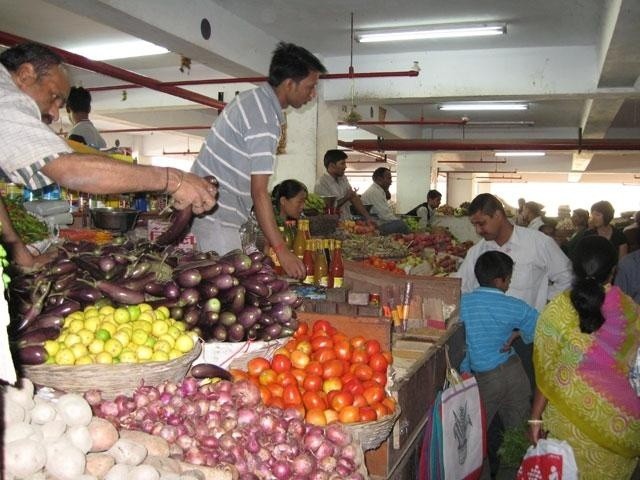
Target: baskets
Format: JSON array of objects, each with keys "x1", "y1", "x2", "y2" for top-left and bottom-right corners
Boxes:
[
  {"x1": 17, "y1": 336, "x2": 286, "y2": 400},
  {"x1": 336, "y1": 401, "x2": 401, "y2": 452}
]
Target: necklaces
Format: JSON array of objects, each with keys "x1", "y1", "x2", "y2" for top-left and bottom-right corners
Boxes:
[{"x1": 73, "y1": 118, "x2": 90, "y2": 126}]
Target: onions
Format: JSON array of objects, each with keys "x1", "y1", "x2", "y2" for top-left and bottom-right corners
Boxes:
[{"x1": 83, "y1": 376, "x2": 372, "y2": 480}]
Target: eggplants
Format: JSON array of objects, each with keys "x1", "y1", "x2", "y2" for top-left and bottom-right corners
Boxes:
[
  {"x1": 153, "y1": 176, "x2": 220, "y2": 245},
  {"x1": 5, "y1": 234, "x2": 305, "y2": 382}
]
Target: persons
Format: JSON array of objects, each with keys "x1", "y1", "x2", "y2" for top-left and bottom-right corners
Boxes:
[
  {"x1": 0, "y1": 42, "x2": 219, "y2": 268},
  {"x1": 271, "y1": 177, "x2": 310, "y2": 224},
  {"x1": 360, "y1": 167, "x2": 392, "y2": 219},
  {"x1": 527, "y1": 237, "x2": 640, "y2": 478},
  {"x1": 65, "y1": 84, "x2": 106, "y2": 152},
  {"x1": 406, "y1": 189, "x2": 442, "y2": 227},
  {"x1": 386, "y1": 190, "x2": 392, "y2": 206},
  {"x1": 312, "y1": 148, "x2": 371, "y2": 225},
  {"x1": 187, "y1": 38, "x2": 328, "y2": 280},
  {"x1": 518, "y1": 198, "x2": 639, "y2": 304},
  {"x1": 454, "y1": 192, "x2": 573, "y2": 479}
]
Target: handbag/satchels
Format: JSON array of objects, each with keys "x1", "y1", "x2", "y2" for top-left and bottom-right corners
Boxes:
[{"x1": 421, "y1": 368, "x2": 486, "y2": 480}]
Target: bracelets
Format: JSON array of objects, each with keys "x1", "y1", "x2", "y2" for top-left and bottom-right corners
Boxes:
[
  {"x1": 166, "y1": 167, "x2": 183, "y2": 196},
  {"x1": 159, "y1": 167, "x2": 170, "y2": 195},
  {"x1": 273, "y1": 243, "x2": 287, "y2": 252}
]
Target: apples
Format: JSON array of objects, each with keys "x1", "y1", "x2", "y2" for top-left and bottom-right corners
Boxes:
[
  {"x1": 344, "y1": 220, "x2": 375, "y2": 234},
  {"x1": 394, "y1": 232, "x2": 473, "y2": 272}
]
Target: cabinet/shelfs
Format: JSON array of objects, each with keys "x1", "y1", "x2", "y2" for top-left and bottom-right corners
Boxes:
[{"x1": 362, "y1": 316, "x2": 467, "y2": 480}]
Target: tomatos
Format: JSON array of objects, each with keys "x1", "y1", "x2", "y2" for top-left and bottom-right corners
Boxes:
[{"x1": 230, "y1": 319, "x2": 396, "y2": 425}]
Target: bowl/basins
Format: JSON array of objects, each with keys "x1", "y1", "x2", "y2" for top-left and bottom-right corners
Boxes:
[
  {"x1": 322, "y1": 196, "x2": 339, "y2": 208},
  {"x1": 86, "y1": 207, "x2": 139, "y2": 231},
  {"x1": 351, "y1": 203, "x2": 373, "y2": 214}
]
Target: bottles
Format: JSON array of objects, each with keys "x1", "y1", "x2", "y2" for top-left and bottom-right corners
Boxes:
[
  {"x1": 1, "y1": 153, "x2": 171, "y2": 213},
  {"x1": 240, "y1": 219, "x2": 346, "y2": 292},
  {"x1": 378, "y1": 280, "x2": 412, "y2": 337}
]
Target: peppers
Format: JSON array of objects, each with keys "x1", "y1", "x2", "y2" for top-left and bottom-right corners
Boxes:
[{"x1": 0, "y1": 184, "x2": 55, "y2": 246}]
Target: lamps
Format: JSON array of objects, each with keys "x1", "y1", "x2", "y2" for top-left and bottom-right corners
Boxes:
[
  {"x1": 433, "y1": 99, "x2": 531, "y2": 113},
  {"x1": 349, "y1": 21, "x2": 509, "y2": 43}
]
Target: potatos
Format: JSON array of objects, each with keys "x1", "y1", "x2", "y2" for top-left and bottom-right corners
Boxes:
[{"x1": 2, "y1": 376, "x2": 206, "y2": 479}]
